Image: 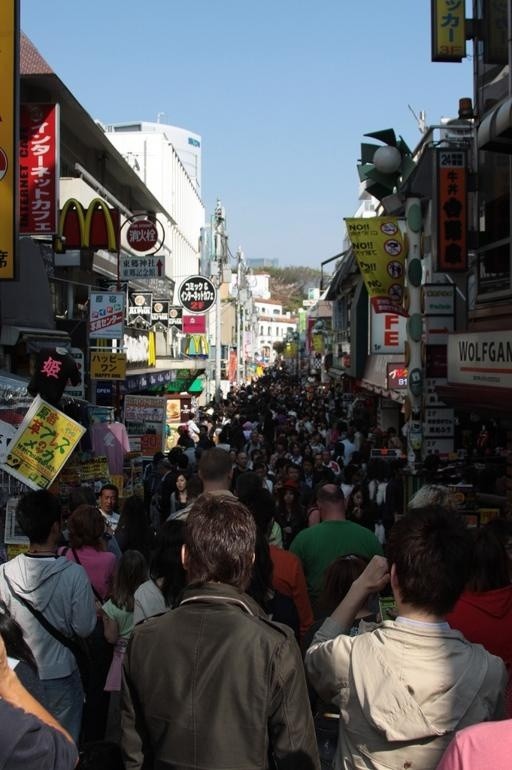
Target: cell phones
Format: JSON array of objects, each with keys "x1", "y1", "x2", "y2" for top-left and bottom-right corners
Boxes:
[{"x1": 378, "y1": 580, "x2": 398, "y2": 622}]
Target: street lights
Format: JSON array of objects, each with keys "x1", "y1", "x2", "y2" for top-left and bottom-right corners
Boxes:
[{"x1": 356, "y1": 127, "x2": 422, "y2": 469}]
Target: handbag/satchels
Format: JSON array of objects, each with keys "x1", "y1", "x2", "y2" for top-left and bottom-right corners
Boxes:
[{"x1": 68, "y1": 616, "x2": 114, "y2": 690}]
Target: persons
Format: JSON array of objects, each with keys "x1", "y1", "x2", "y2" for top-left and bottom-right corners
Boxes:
[{"x1": 0, "y1": 362, "x2": 512, "y2": 769}]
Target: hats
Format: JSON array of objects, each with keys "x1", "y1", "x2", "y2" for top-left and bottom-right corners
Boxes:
[{"x1": 278, "y1": 479, "x2": 299, "y2": 491}]
